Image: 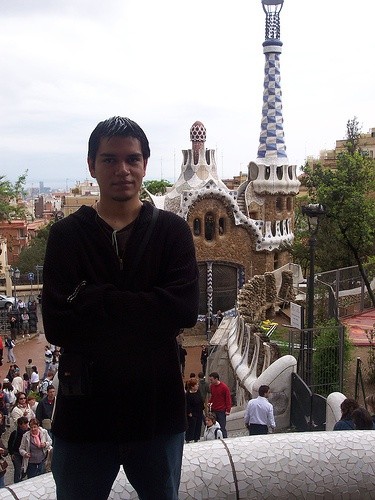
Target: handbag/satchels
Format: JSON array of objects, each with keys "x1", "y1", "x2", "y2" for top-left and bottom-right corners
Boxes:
[
  {"x1": 41, "y1": 419, "x2": 51, "y2": 430},
  {"x1": 1, "y1": 460, "x2": 8, "y2": 470}
]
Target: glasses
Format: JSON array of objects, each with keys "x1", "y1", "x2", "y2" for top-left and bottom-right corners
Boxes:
[{"x1": 19, "y1": 397, "x2": 26, "y2": 400}]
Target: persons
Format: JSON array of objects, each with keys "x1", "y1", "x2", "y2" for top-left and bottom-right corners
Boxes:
[
  {"x1": 208, "y1": 372, "x2": 231, "y2": 438},
  {"x1": 216, "y1": 310, "x2": 223, "y2": 326},
  {"x1": 40, "y1": 117, "x2": 200, "y2": 500},
  {"x1": 10, "y1": 392, "x2": 35, "y2": 431},
  {"x1": 201, "y1": 345, "x2": 207, "y2": 363},
  {"x1": 334, "y1": 399, "x2": 375, "y2": 431},
  {"x1": 7, "y1": 417, "x2": 30, "y2": 484},
  {"x1": 8, "y1": 294, "x2": 38, "y2": 344},
  {"x1": 0, "y1": 359, "x2": 40, "y2": 489},
  {"x1": 203, "y1": 412, "x2": 223, "y2": 441},
  {"x1": 36, "y1": 385, "x2": 57, "y2": 446},
  {"x1": 185, "y1": 372, "x2": 196, "y2": 390},
  {"x1": 0, "y1": 334, "x2": 16, "y2": 366},
  {"x1": 185, "y1": 377, "x2": 205, "y2": 443},
  {"x1": 205, "y1": 313, "x2": 215, "y2": 339},
  {"x1": 198, "y1": 373, "x2": 210, "y2": 403},
  {"x1": 42, "y1": 344, "x2": 60, "y2": 390},
  {"x1": 244, "y1": 385, "x2": 275, "y2": 435},
  {"x1": 19, "y1": 418, "x2": 53, "y2": 479},
  {"x1": 178, "y1": 344, "x2": 187, "y2": 376}
]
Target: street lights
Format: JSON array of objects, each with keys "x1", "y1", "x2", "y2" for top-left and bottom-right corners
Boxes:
[
  {"x1": 8, "y1": 266, "x2": 21, "y2": 310},
  {"x1": 301, "y1": 201, "x2": 330, "y2": 388}
]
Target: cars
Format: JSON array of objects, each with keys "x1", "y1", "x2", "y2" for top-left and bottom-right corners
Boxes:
[{"x1": 0, "y1": 295, "x2": 25, "y2": 310}]
